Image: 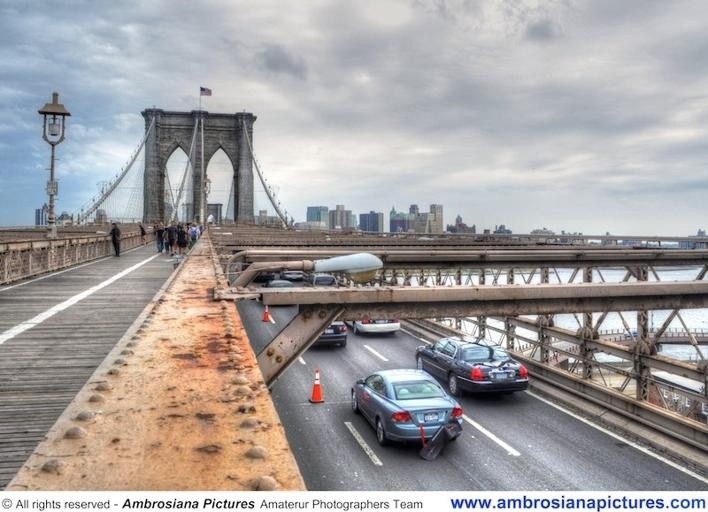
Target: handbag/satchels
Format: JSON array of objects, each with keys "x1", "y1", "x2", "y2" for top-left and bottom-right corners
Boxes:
[{"x1": 115, "y1": 235, "x2": 121, "y2": 243}]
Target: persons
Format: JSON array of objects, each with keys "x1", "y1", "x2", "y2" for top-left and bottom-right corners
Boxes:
[
  {"x1": 109, "y1": 222, "x2": 122, "y2": 257},
  {"x1": 153, "y1": 220, "x2": 204, "y2": 257},
  {"x1": 139, "y1": 224, "x2": 147, "y2": 241}
]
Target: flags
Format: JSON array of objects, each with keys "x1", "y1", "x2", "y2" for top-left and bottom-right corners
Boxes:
[{"x1": 200, "y1": 86, "x2": 212, "y2": 96}]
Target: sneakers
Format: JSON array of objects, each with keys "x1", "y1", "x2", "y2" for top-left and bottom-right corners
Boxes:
[{"x1": 158, "y1": 250, "x2": 176, "y2": 256}]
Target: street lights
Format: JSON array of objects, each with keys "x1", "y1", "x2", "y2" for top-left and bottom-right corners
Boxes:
[
  {"x1": 200, "y1": 171, "x2": 213, "y2": 233},
  {"x1": 36, "y1": 86, "x2": 72, "y2": 237},
  {"x1": 700, "y1": 321, "x2": 707, "y2": 324},
  {"x1": 180, "y1": 201, "x2": 185, "y2": 226}
]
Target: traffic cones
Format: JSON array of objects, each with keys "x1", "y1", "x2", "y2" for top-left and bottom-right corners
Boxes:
[
  {"x1": 306, "y1": 366, "x2": 327, "y2": 404},
  {"x1": 262, "y1": 305, "x2": 270, "y2": 322}
]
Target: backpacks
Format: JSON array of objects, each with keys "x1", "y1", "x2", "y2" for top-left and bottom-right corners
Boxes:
[{"x1": 190, "y1": 227, "x2": 197, "y2": 241}]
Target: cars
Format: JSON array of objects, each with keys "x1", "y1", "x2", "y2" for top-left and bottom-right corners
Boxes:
[
  {"x1": 310, "y1": 319, "x2": 348, "y2": 350},
  {"x1": 241, "y1": 263, "x2": 337, "y2": 307},
  {"x1": 349, "y1": 367, "x2": 465, "y2": 449},
  {"x1": 623, "y1": 328, "x2": 638, "y2": 338},
  {"x1": 411, "y1": 334, "x2": 531, "y2": 400},
  {"x1": 344, "y1": 318, "x2": 401, "y2": 337}
]
